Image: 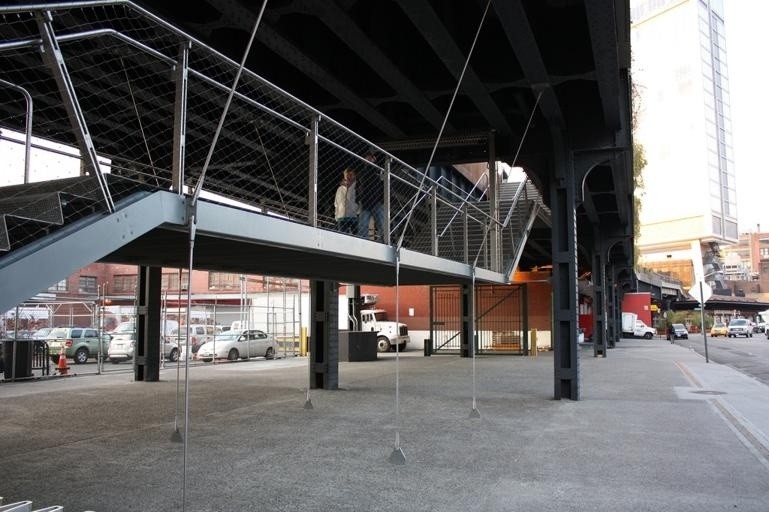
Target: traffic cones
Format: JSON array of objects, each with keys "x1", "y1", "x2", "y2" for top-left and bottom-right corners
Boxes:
[{"x1": 55, "y1": 346, "x2": 70, "y2": 375}]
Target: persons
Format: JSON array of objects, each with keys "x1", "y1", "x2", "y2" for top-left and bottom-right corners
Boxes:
[
  {"x1": 669, "y1": 322, "x2": 676, "y2": 344},
  {"x1": 334, "y1": 152, "x2": 385, "y2": 243}
]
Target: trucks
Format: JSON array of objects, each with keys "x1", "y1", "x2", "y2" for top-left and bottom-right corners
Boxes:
[
  {"x1": 249, "y1": 294, "x2": 410, "y2": 353},
  {"x1": 605, "y1": 312, "x2": 657, "y2": 339},
  {"x1": 757, "y1": 311, "x2": 765, "y2": 332}
]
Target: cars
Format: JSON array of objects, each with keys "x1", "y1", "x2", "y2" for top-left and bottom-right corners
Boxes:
[
  {"x1": 1, "y1": 322, "x2": 278, "y2": 364},
  {"x1": 711, "y1": 318, "x2": 757, "y2": 338},
  {"x1": 667, "y1": 323, "x2": 689, "y2": 340}
]
